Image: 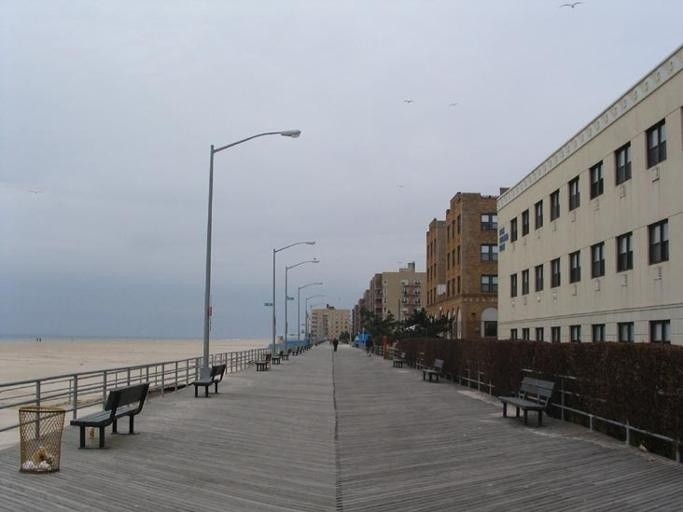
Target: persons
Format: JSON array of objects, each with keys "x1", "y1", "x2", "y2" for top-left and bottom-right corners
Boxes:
[
  {"x1": 333, "y1": 338, "x2": 338, "y2": 351},
  {"x1": 366, "y1": 336, "x2": 373, "y2": 357}
]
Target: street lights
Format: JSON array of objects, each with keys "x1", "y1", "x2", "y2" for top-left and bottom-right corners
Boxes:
[
  {"x1": 284, "y1": 259, "x2": 320, "y2": 353},
  {"x1": 305, "y1": 294, "x2": 325, "y2": 340},
  {"x1": 271, "y1": 241, "x2": 315, "y2": 353},
  {"x1": 202, "y1": 128, "x2": 302, "y2": 375},
  {"x1": 296, "y1": 282, "x2": 323, "y2": 347}
]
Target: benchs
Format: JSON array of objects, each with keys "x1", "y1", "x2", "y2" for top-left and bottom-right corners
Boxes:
[
  {"x1": 272, "y1": 345, "x2": 310, "y2": 364},
  {"x1": 498, "y1": 376, "x2": 555, "y2": 426},
  {"x1": 70, "y1": 381, "x2": 150, "y2": 448},
  {"x1": 193, "y1": 364, "x2": 226, "y2": 397},
  {"x1": 422, "y1": 359, "x2": 444, "y2": 382},
  {"x1": 393, "y1": 352, "x2": 407, "y2": 367},
  {"x1": 255, "y1": 354, "x2": 271, "y2": 371}
]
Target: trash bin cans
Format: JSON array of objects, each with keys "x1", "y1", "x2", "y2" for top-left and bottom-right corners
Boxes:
[{"x1": 19, "y1": 406, "x2": 66, "y2": 474}]
